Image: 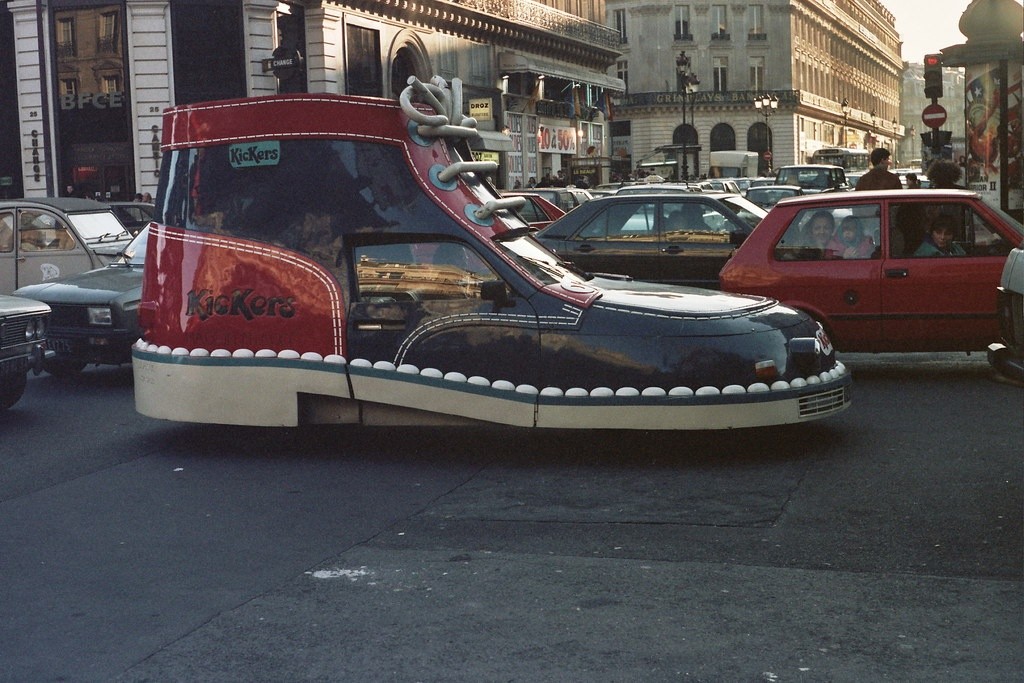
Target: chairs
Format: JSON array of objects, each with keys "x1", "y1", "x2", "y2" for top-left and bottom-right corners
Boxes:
[
  {"x1": 664, "y1": 210, "x2": 686, "y2": 234},
  {"x1": 889, "y1": 226, "x2": 907, "y2": 259}
]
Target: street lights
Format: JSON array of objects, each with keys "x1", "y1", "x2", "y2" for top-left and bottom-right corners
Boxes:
[
  {"x1": 910, "y1": 125, "x2": 916, "y2": 159},
  {"x1": 892, "y1": 116, "x2": 898, "y2": 162},
  {"x1": 842, "y1": 98, "x2": 851, "y2": 148},
  {"x1": 754, "y1": 89, "x2": 779, "y2": 174},
  {"x1": 871, "y1": 108, "x2": 879, "y2": 149},
  {"x1": 675, "y1": 50, "x2": 700, "y2": 181}
]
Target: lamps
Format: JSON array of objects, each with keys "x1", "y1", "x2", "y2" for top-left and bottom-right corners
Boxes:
[
  {"x1": 537, "y1": 75, "x2": 545, "y2": 79},
  {"x1": 501, "y1": 73, "x2": 509, "y2": 79}
]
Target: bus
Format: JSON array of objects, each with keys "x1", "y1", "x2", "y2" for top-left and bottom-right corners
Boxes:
[
  {"x1": 807, "y1": 148, "x2": 874, "y2": 186},
  {"x1": 909, "y1": 159, "x2": 923, "y2": 170}
]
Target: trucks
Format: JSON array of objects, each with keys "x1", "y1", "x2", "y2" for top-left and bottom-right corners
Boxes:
[{"x1": 709, "y1": 150, "x2": 759, "y2": 178}]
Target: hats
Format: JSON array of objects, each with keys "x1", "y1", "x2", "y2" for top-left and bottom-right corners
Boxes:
[{"x1": 929, "y1": 215, "x2": 958, "y2": 242}]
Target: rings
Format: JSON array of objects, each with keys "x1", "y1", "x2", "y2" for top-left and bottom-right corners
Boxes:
[{"x1": 853, "y1": 251, "x2": 855, "y2": 254}]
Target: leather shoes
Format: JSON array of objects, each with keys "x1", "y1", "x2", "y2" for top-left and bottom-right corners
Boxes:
[{"x1": 134, "y1": 75, "x2": 853, "y2": 429}]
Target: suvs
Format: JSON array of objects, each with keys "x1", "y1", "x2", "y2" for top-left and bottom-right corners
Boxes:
[{"x1": 775, "y1": 164, "x2": 856, "y2": 195}]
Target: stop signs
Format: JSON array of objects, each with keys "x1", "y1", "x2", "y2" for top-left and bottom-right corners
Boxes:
[
  {"x1": 922, "y1": 104, "x2": 947, "y2": 128},
  {"x1": 764, "y1": 151, "x2": 771, "y2": 161}
]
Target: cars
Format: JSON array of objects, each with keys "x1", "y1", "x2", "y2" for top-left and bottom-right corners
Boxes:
[
  {"x1": 498, "y1": 178, "x2": 804, "y2": 291},
  {"x1": 718, "y1": 189, "x2": 1024, "y2": 354},
  {"x1": 889, "y1": 168, "x2": 931, "y2": 188},
  {"x1": 0, "y1": 198, "x2": 156, "y2": 409}
]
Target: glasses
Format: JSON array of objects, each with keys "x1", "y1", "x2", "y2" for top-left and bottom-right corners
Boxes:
[{"x1": 842, "y1": 225, "x2": 857, "y2": 233}]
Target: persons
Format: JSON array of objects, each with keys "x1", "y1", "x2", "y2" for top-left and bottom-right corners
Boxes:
[
  {"x1": 576, "y1": 175, "x2": 591, "y2": 188},
  {"x1": 525, "y1": 177, "x2": 551, "y2": 189},
  {"x1": 681, "y1": 203, "x2": 713, "y2": 230},
  {"x1": 64, "y1": 186, "x2": 78, "y2": 197},
  {"x1": 135, "y1": 193, "x2": 143, "y2": 202},
  {"x1": 925, "y1": 158, "x2": 967, "y2": 189},
  {"x1": 912, "y1": 213, "x2": 967, "y2": 256},
  {"x1": 780, "y1": 210, "x2": 874, "y2": 259},
  {"x1": 668, "y1": 168, "x2": 673, "y2": 180},
  {"x1": 420, "y1": 244, "x2": 481, "y2": 316},
  {"x1": 609, "y1": 167, "x2": 655, "y2": 183},
  {"x1": 48, "y1": 220, "x2": 74, "y2": 249},
  {"x1": 905, "y1": 173, "x2": 921, "y2": 189},
  {"x1": 689, "y1": 175, "x2": 696, "y2": 180},
  {"x1": 7, "y1": 222, "x2": 47, "y2": 251},
  {"x1": 699, "y1": 173, "x2": 707, "y2": 180},
  {"x1": 546, "y1": 170, "x2": 568, "y2": 187},
  {"x1": 853, "y1": 147, "x2": 903, "y2": 217},
  {"x1": 143, "y1": 192, "x2": 152, "y2": 203},
  {"x1": 514, "y1": 180, "x2": 522, "y2": 189}
]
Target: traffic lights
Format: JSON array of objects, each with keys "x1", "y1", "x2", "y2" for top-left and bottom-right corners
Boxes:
[{"x1": 924, "y1": 55, "x2": 943, "y2": 98}]
